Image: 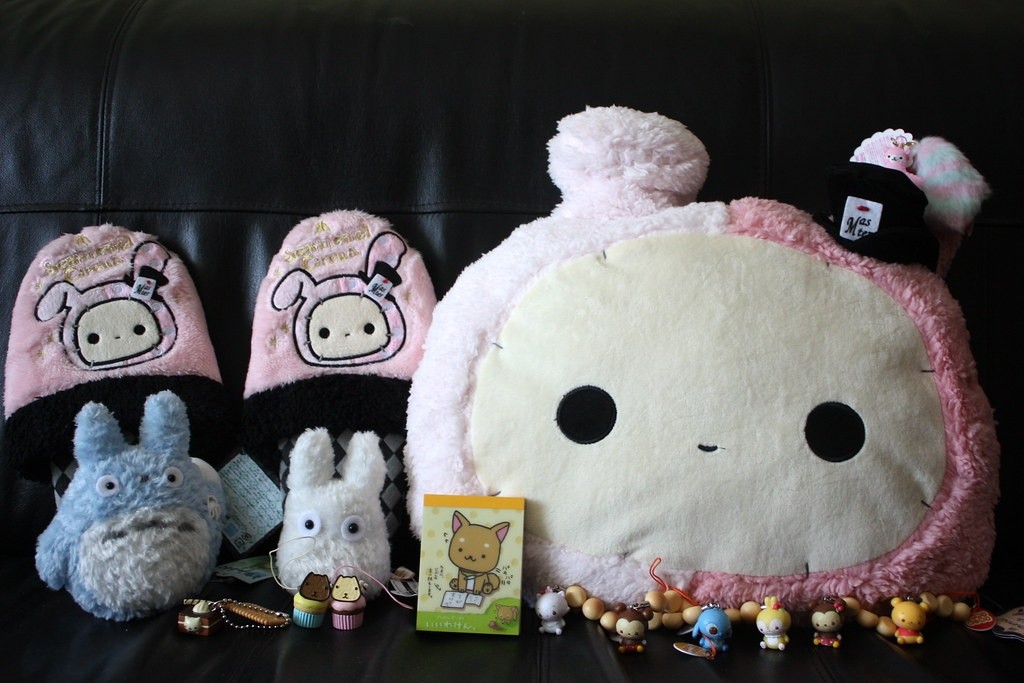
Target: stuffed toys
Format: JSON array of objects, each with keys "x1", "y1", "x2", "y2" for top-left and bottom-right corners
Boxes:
[
  {"x1": 403, "y1": 105, "x2": 995, "y2": 616},
  {"x1": 280, "y1": 429, "x2": 391, "y2": 595},
  {"x1": 882, "y1": 137, "x2": 925, "y2": 187},
  {"x1": 33, "y1": 390, "x2": 225, "y2": 622}
]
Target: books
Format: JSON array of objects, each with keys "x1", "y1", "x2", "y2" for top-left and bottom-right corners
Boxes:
[{"x1": 416, "y1": 494, "x2": 526, "y2": 635}]
